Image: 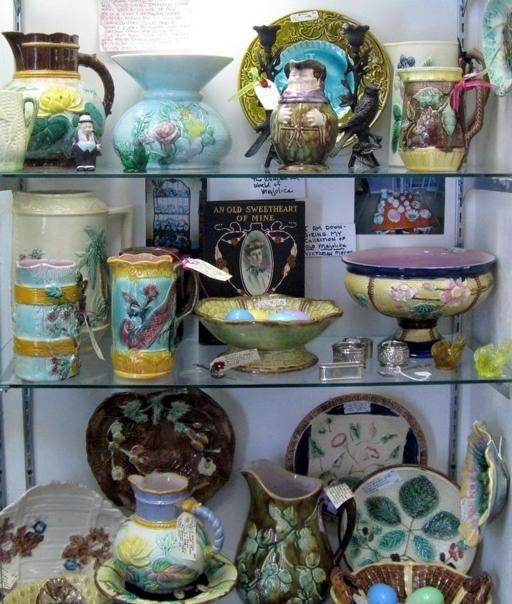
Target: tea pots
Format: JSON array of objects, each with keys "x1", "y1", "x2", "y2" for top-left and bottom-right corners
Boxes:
[
  {"x1": 8, "y1": 189, "x2": 133, "y2": 348},
  {"x1": 1, "y1": 32, "x2": 114, "y2": 166},
  {"x1": 383, "y1": 40, "x2": 491, "y2": 171}
]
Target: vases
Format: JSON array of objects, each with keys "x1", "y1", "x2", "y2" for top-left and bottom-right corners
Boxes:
[{"x1": 1, "y1": 165, "x2": 512, "y2": 389}]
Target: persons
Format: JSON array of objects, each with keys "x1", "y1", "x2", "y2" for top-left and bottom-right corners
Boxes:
[
  {"x1": 270, "y1": 59, "x2": 338, "y2": 172},
  {"x1": 243, "y1": 238, "x2": 271, "y2": 294},
  {"x1": 72, "y1": 110, "x2": 102, "y2": 169}
]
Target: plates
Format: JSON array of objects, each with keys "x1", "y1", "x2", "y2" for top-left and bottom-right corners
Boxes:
[
  {"x1": 482, "y1": 0, "x2": 512, "y2": 95},
  {"x1": 236, "y1": 9, "x2": 394, "y2": 148},
  {"x1": 0, "y1": 386, "x2": 510, "y2": 603}
]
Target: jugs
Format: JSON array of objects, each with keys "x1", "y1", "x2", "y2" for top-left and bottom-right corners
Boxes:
[
  {"x1": 111, "y1": 470, "x2": 226, "y2": 592},
  {"x1": 235, "y1": 457, "x2": 358, "y2": 601}
]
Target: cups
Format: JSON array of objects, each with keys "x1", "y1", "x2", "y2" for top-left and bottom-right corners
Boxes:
[
  {"x1": 14, "y1": 254, "x2": 200, "y2": 382},
  {"x1": 0, "y1": 91, "x2": 39, "y2": 174}
]
